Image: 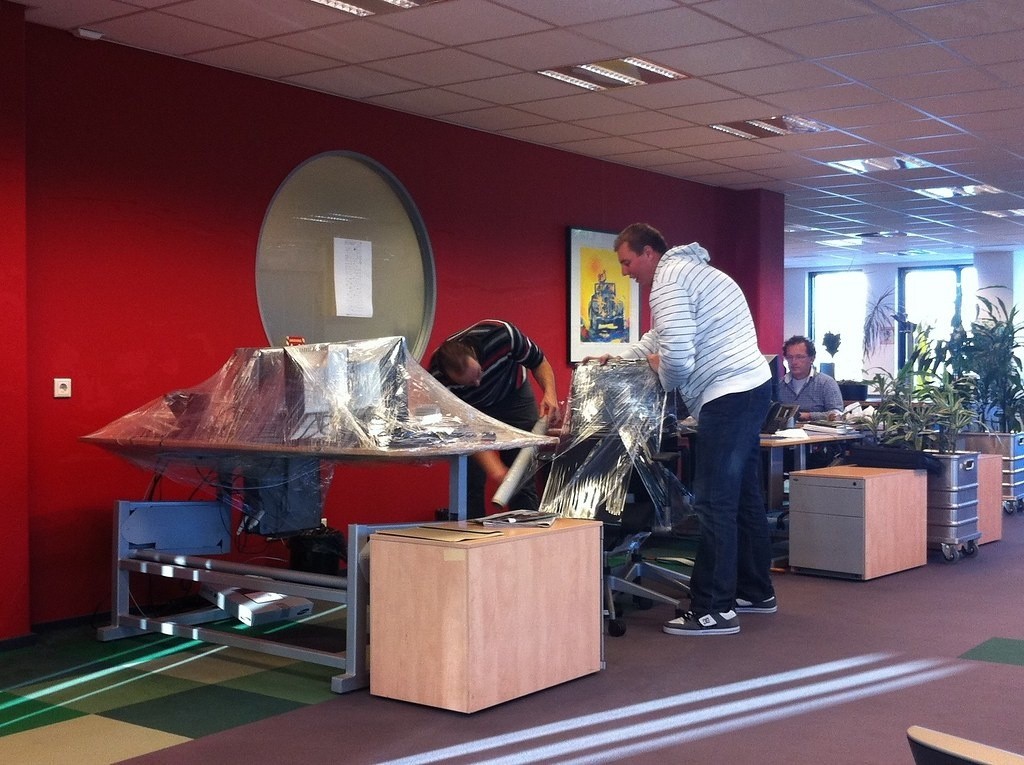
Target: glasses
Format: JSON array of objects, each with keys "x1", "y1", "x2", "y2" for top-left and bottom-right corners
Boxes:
[{"x1": 786, "y1": 356, "x2": 810, "y2": 361}]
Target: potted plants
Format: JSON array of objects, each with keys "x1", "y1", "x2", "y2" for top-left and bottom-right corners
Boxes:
[
  {"x1": 849, "y1": 359, "x2": 1002, "y2": 469},
  {"x1": 836, "y1": 379, "x2": 867, "y2": 400},
  {"x1": 820, "y1": 331, "x2": 842, "y2": 379}
]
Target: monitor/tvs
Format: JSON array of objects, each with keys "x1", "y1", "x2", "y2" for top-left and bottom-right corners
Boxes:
[
  {"x1": 761, "y1": 353, "x2": 781, "y2": 403},
  {"x1": 228, "y1": 337, "x2": 410, "y2": 444}
]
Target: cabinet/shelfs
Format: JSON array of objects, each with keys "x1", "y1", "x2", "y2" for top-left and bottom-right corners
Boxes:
[
  {"x1": 368, "y1": 517, "x2": 608, "y2": 715},
  {"x1": 788, "y1": 464, "x2": 927, "y2": 583},
  {"x1": 927, "y1": 453, "x2": 1003, "y2": 551}
]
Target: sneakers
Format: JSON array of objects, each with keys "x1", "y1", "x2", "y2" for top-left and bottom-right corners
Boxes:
[
  {"x1": 663, "y1": 607, "x2": 740, "y2": 635},
  {"x1": 734, "y1": 592, "x2": 777, "y2": 613}
]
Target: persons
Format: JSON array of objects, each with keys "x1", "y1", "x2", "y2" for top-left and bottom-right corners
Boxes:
[
  {"x1": 582, "y1": 223, "x2": 778, "y2": 635},
  {"x1": 776, "y1": 335, "x2": 843, "y2": 421},
  {"x1": 427, "y1": 319, "x2": 561, "y2": 520}
]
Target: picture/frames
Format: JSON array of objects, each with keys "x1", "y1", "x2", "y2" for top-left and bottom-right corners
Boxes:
[{"x1": 565, "y1": 223, "x2": 643, "y2": 367}]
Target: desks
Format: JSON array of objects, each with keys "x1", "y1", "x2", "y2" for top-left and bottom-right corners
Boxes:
[
  {"x1": 546, "y1": 429, "x2": 866, "y2": 542},
  {"x1": 843, "y1": 396, "x2": 933, "y2": 414},
  {"x1": 78, "y1": 435, "x2": 560, "y2": 695}
]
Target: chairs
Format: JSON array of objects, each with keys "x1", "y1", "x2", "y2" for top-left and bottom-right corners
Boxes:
[{"x1": 508, "y1": 359, "x2": 691, "y2": 638}]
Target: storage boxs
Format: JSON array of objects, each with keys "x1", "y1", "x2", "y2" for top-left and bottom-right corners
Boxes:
[{"x1": 198, "y1": 583, "x2": 313, "y2": 627}]
